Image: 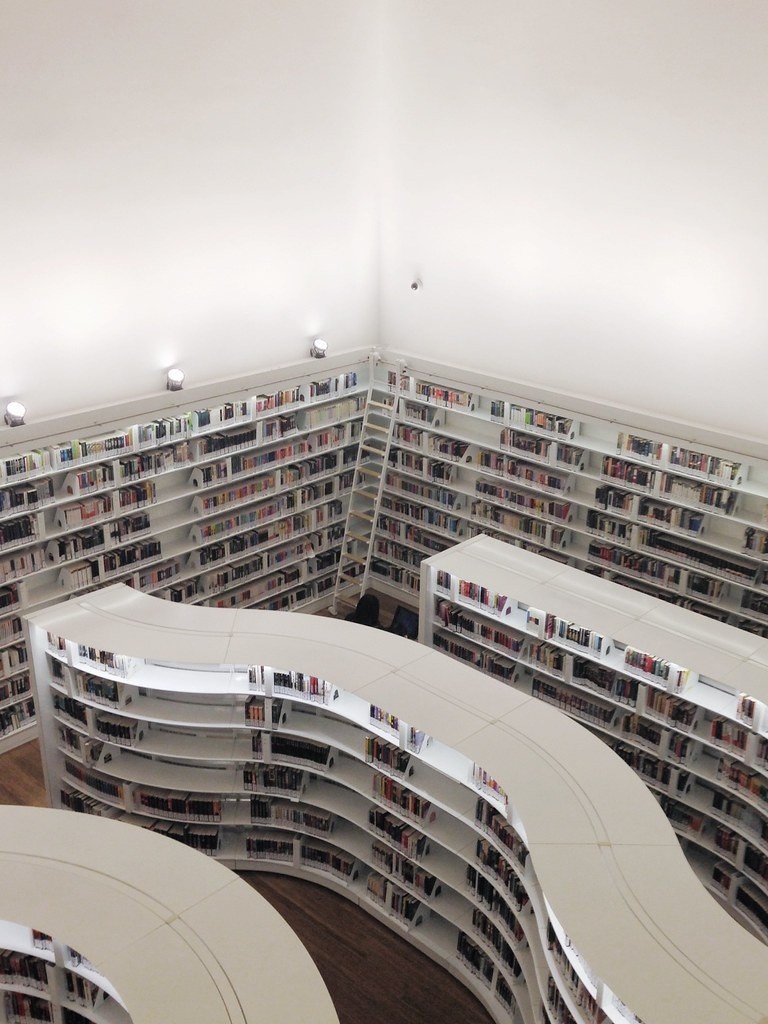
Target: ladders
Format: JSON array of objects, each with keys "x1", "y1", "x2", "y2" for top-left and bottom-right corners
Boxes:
[{"x1": 326, "y1": 353, "x2": 407, "y2": 617}]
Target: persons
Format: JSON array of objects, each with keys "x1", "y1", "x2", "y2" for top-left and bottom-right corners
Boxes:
[{"x1": 346, "y1": 594, "x2": 387, "y2": 630}]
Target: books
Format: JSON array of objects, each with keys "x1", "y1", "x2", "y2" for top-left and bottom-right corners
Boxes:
[{"x1": 0, "y1": 366, "x2": 767, "y2": 1024}]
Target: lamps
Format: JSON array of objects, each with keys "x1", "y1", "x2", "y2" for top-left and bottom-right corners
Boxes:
[
  {"x1": 4, "y1": 401, "x2": 27, "y2": 427},
  {"x1": 167, "y1": 368, "x2": 184, "y2": 391},
  {"x1": 309, "y1": 338, "x2": 328, "y2": 358}
]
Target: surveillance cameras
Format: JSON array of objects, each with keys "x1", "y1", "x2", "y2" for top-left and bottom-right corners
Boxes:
[{"x1": 411, "y1": 279, "x2": 423, "y2": 291}]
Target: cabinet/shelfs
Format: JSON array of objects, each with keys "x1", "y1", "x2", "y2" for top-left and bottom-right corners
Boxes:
[
  {"x1": 418, "y1": 534, "x2": 767, "y2": 953},
  {"x1": 0, "y1": 346, "x2": 768, "y2": 756},
  {"x1": 0, "y1": 805, "x2": 342, "y2": 1024},
  {"x1": 22, "y1": 582, "x2": 768, "y2": 1024}
]
